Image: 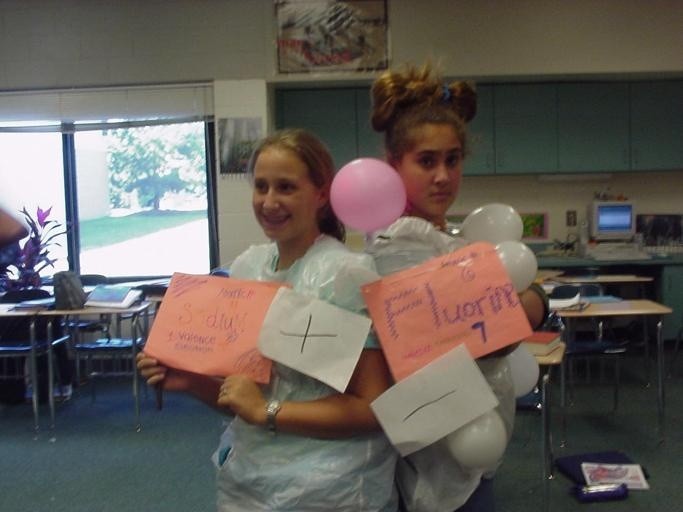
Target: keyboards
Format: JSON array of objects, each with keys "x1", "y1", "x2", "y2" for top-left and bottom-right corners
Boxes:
[{"x1": 592, "y1": 250, "x2": 652, "y2": 261}]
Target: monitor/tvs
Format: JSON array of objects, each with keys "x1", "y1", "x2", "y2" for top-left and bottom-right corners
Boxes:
[{"x1": 588, "y1": 201, "x2": 636, "y2": 240}]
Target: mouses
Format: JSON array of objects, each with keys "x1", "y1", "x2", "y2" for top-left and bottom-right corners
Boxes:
[{"x1": 657, "y1": 250, "x2": 668, "y2": 258}]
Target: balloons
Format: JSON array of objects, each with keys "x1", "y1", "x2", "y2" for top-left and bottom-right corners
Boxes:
[
  {"x1": 458, "y1": 203, "x2": 524, "y2": 247},
  {"x1": 503, "y1": 347, "x2": 540, "y2": 399},
  {"x1": 448, "y1": 409, "x2": 508, "y2": 472},
  {"x1": 494, "y1": 240, "x2": 538, "y2": 295},
  {"x1": 331, "y1": 156, "x2": 406, "y2": 234}
]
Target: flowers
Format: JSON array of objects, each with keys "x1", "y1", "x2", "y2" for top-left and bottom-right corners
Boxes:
[{"x1": 0, "y1": 205, "x2": 74, "y2": 292}]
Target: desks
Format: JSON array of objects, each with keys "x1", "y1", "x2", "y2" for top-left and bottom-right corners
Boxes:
[{"x1": 517, "y1": 250, "x2": 683, "y2": 512}]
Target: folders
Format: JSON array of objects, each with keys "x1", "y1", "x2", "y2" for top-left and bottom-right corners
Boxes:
[{"x1": 83, "y1": 288, "x2": 143, "y2": 309}]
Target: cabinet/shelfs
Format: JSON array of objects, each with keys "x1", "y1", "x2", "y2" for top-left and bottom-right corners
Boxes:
[{"x1": 275, "y1": 80, "x2": 683, "y2": 177}]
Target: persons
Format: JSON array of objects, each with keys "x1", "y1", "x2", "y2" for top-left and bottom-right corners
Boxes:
[
  {"x1": 135, "y1": 130, "x2": 396, "y2": 512},
  {"x1": 1, "y1": 204, "x2": 28, "y2": 246},
  {"x1": 366, "y1": 58, "x2": 549, "y2": 512}
]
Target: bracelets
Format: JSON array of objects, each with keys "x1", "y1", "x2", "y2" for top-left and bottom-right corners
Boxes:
[{"x1": 265, "y1": 399, "x2": 282, "y2": 435}]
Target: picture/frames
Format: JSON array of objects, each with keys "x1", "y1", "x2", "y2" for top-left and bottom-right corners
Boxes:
[{"x1": 275, "y1": 0, "x2": 389, "y2": 73}]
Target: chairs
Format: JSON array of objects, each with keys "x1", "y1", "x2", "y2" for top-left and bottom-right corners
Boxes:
[{"x1": 0, "y1": 275, "x2": 162, "y2": 442}]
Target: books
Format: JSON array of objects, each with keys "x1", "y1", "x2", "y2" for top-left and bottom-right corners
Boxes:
[{"x1": 521, "y1": 332, "x2": 561, "y2": 357}]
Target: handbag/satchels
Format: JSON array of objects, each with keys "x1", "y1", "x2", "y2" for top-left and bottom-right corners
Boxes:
[{"x1": 54, "y1": 271, "x2": 87, "y2": 309}]
